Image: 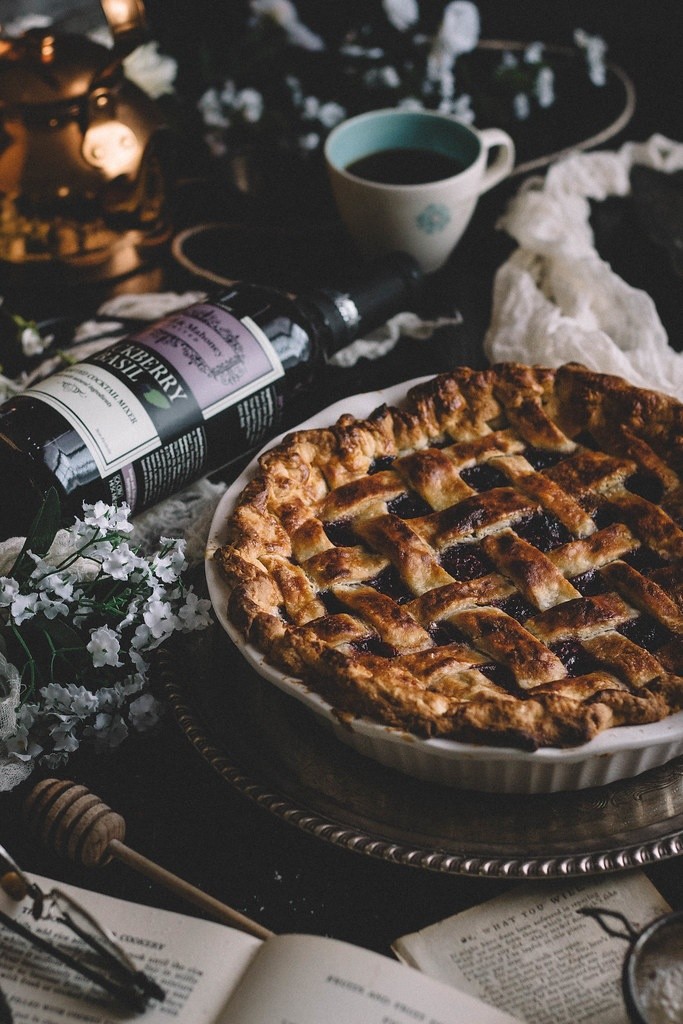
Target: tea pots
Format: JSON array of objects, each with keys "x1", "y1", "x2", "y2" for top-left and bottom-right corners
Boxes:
[{"x1": 1, "y1": 0, "x2": 184, "y2": 268}]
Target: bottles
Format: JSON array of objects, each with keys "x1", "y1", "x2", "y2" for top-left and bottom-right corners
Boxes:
[{"x1": 0, "y1": 253, "x2": 423, "y2": 537}]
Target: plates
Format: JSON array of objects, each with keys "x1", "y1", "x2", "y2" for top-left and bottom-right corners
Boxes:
[{"x1": 139, "y1": 500, "x2": 683, "y2": 880}]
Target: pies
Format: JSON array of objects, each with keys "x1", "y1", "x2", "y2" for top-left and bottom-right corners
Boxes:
[{"x1": 214, "y1": 361, "x2": 683, "y2": 754}]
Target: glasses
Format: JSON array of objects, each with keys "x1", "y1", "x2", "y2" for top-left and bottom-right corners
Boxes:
[{"x1": 0, "y1": 847, "x2": 164, "y2": 1014}]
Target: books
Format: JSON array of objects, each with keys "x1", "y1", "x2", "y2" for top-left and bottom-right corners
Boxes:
[
  {"x1": 391, "y1": 865, "x2": 674, "y2": 1024},
  {"x1": 0, "y1": 870, "x2": 532, "y2": 1024}
]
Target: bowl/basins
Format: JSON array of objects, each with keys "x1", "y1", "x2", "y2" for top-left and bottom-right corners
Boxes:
[{"x1": 203, "y1": 371, "x2": 682, "y2": 796}]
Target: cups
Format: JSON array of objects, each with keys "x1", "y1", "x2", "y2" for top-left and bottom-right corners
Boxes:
[{"x1": 323, "y1": 109, "x2": 515, "y2": 277}]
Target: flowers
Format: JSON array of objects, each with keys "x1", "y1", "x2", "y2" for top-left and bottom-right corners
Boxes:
[
  {"x1": 120, "y1": 2, "x2": 612, "y2": 197},
  {"x1": 14, "y1": 309, "x2": 156, "y2": 392},
  {"x1": 1, "y1": 498, "x2": 215, "y2": 793}
]
